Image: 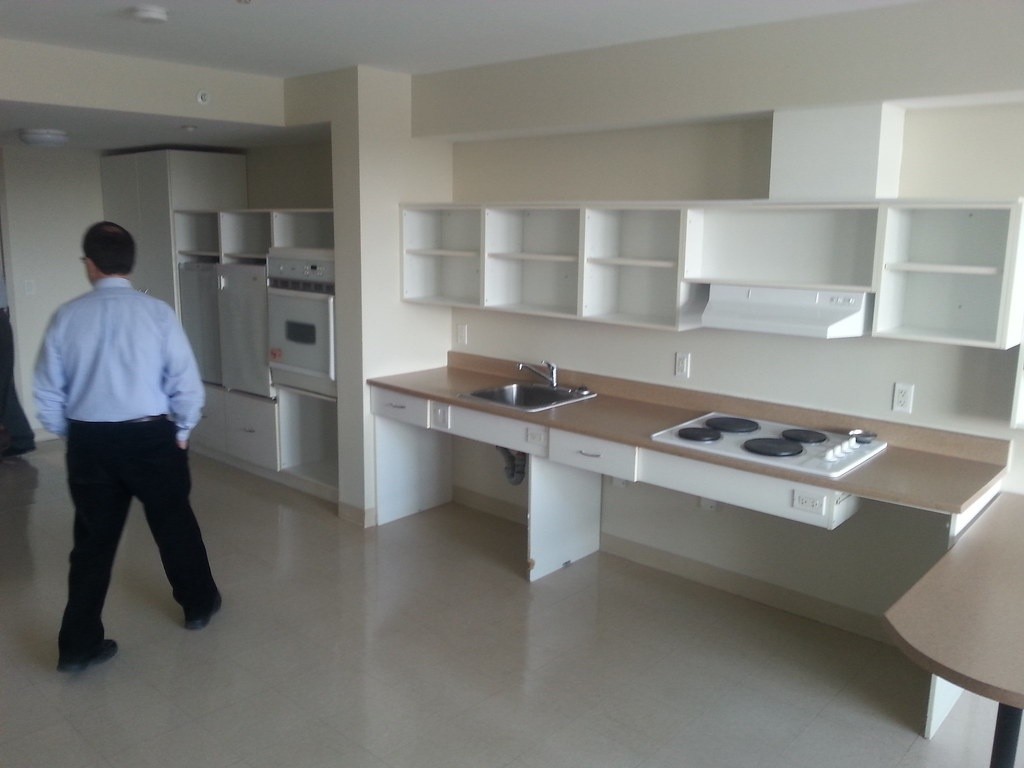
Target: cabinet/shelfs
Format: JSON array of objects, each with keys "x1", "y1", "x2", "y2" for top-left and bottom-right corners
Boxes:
[{"x1": 164, "y1": 95, "x2": 1024, "y2": 742}]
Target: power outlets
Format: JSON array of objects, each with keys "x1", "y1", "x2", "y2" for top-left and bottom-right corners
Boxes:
[
  {"x1": 457, "y1": 324, "x2": 468, "y2": 345},
  {"x1": 892, "y1": 381, "x2": 915, "y2": 414},
  {"x1": 792, "y1": 489, "x2": 826, "y2": 516},
  {"x1": 674, "y1": 351, "x2": 690, "y2": 379}
]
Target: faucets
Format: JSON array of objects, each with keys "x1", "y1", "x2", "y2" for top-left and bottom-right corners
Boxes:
[{"x1": 518, "y1": 360, "x2": 557, "y2": 387}]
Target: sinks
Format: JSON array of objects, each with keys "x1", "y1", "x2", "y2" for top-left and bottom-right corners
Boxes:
[{"x1": 472, "y1": 384, "x2": 572, "y2": 406}]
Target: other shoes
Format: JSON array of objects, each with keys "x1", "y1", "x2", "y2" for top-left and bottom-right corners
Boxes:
[
  {"x1": 184, "y1": 591, "x2": 221, "y2": 629},
  {"x1": 56, "y1": 639, "x2": 118, "y2": 672}
]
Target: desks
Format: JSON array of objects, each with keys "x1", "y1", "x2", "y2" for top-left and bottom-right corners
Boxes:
[{"x1": 883, "y1": 491, "x2": 1024, "y2": 768}]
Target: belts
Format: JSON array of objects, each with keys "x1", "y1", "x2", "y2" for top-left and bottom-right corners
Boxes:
[{"x1": 129, "y1": 415, "x2": 164, "y2": 423}]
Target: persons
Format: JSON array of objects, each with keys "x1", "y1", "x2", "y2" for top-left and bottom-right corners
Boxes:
[{"x1": 34, "y1": 222, "x2": 221, "y2": 672}]
